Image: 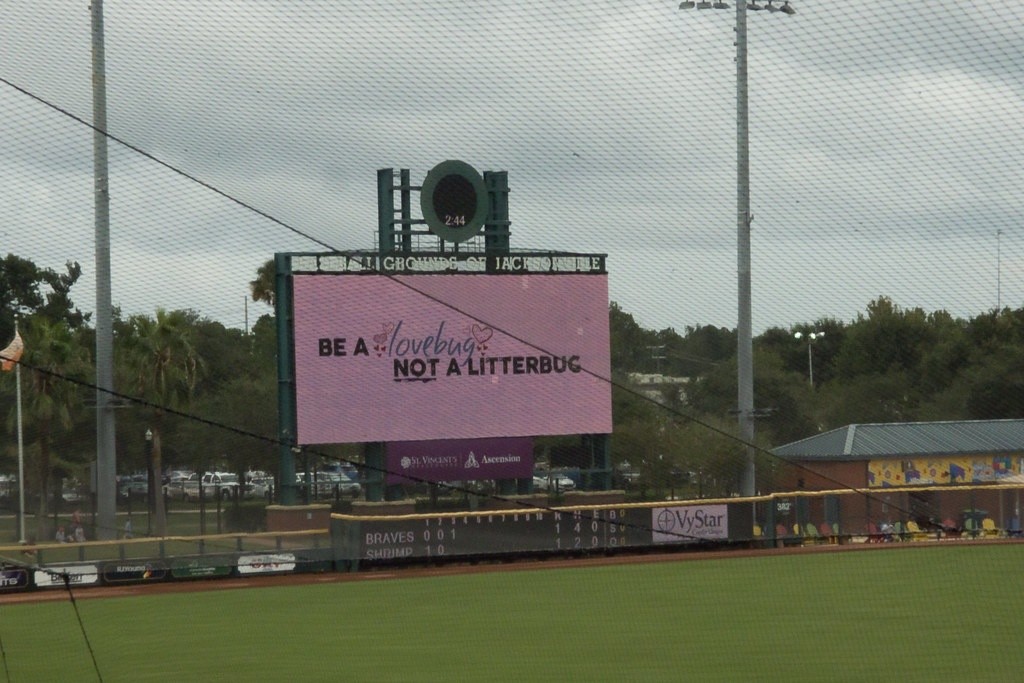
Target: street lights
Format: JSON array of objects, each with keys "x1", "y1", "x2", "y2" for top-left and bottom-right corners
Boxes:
[
  {"x1": 678, "y1": 0, "x2": 798, "y2": 500},
  {"x1": 794, "y1": 330, "x2": 826, "y2": 389}
]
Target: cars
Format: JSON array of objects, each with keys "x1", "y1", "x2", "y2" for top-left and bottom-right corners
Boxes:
[
  {"x1": 61, "y1": 468, "x2": 362, "y2": 506},
  {"x1": 534, "y1": 473, "x2": 576, "y2": 492}
]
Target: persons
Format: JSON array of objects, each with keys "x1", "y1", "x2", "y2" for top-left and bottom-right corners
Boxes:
[
  {"x1": 123, "y1": 518, "x2": 136, "y2": 541},
  {"x1": 55, "y1": 507, "x2": 87, "y2": 544}
]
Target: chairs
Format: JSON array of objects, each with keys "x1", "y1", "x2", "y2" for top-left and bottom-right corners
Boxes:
[
  {"x1": 754, "y1": 521, "x2": 840, "y2": 547},
  {"x1": 866, "y1": 518, "x2": 1022, "y2": 545}
]
